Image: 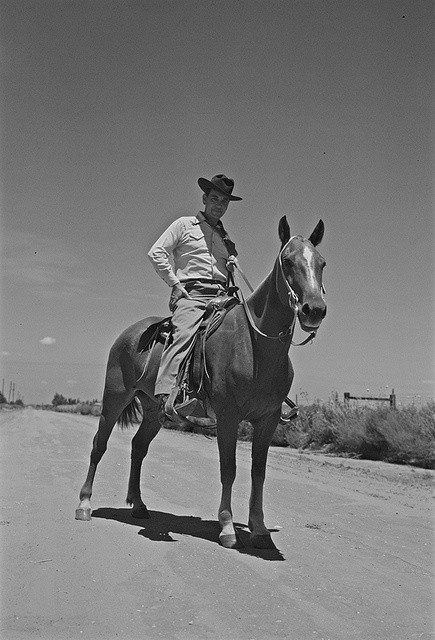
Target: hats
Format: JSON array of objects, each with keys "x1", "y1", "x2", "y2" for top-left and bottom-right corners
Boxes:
[{"x1": 198, "y1": 174, "x2": 243, "y2": 201}]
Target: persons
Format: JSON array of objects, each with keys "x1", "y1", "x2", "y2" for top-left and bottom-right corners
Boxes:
[{"x1": 148, "y1": 174, "x2": 243, "y2": 429}]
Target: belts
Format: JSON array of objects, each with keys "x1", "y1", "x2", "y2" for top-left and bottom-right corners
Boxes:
[{"x1": 199, "y1": 279, "x2": 222, "y2": 285}]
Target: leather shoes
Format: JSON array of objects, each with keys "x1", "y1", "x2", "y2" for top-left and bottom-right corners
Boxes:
[
  {"x1": 158, "y1": 398, "x2": 198, "y2": 424},
  {"x1": 279, "y1": 406, "x2": 299, "y2": 425}
]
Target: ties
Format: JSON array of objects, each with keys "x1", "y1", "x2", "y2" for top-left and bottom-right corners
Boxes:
[{"x1": 209, "y1": 225, "x2": 238, "y2": 259}]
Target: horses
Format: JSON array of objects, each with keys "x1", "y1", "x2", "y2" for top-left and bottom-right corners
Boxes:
[{"x1": 75, "y1": 214, "x2": 326, "y2": 547}]
